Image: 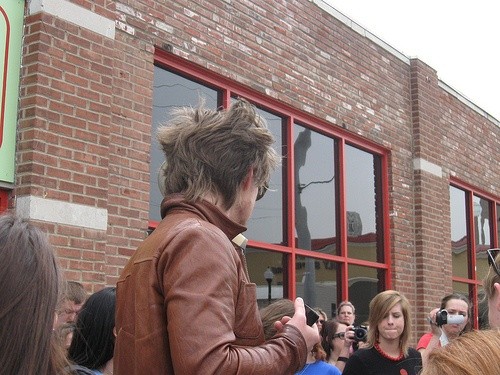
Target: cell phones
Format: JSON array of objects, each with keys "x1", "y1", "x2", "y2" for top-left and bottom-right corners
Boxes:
[{"x1": 304, "y1": 304, "x2": 319, "y2": 327}]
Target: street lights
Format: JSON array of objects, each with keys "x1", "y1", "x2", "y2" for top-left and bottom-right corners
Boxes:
[{"x1": 264, "y1": 268, "x2": 273, "y2": 303}]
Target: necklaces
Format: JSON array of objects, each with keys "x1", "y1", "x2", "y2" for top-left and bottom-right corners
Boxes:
[{"x1": 375, "y1": 342, "x2": 404, "y2": 361}]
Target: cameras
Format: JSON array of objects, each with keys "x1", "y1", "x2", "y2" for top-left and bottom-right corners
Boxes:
[
  {"x1": 436, "y1": 310, "x2": 464, "y2": 325},
  {"x1": 349, "y1": 327, "x2": 368, "y2": 343}
]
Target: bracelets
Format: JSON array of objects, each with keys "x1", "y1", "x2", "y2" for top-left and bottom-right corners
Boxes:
[{"x1": 337, "y1": 357, "x2": 349, "y2": 363}]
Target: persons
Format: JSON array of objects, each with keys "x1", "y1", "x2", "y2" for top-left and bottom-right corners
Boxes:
[
  {"x1": 114, "y1": 97, "x2": 321, "y2": 375},
  {"x1": 54, "y1": 280, "x2": 118, "y2": 375},
  {"x1": 0, "y1": 214, "x2": 77, "y2": 375},
  {"x1": 259, "y1": 295, "x2": 371, "y2": 375},
  {"x1": 341, "y1": 291, "x2": 423, "y2": 375},
  {"x1": 416, "y1": 248, "x2": 500, "y2": 375}
]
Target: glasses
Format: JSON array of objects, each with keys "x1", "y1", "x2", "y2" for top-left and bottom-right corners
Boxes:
[
  {"x1": 486, "y1": 248, "x2": 500, "y2": 277},
  {"x1": 255, "y1": 181, "x2": 269, "y2": 202},
  {"x1": 333, "y1": 332, "x2": 345, "y2": 340}
]
